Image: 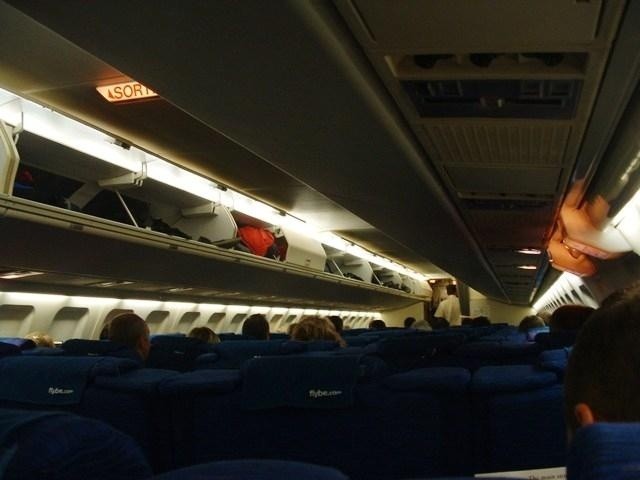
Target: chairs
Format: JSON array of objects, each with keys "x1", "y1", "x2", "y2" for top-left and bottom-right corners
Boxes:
[{"x1": 3, "y1": 322, "x2": 639, "y2": 480}]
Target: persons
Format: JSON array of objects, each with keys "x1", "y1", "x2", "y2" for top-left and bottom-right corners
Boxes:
[
  {"x1": 434, "y1": 283, "x2": 462, "y2": 327},
  {"x1": 516, "y1": 286, "x2": 640, "y2": 446}
]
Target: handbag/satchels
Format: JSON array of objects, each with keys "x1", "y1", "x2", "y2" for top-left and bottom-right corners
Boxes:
[
  {"x1": 343, "y1": 268, "x2": 413, "y2": 295},
  {"x1": 235, "y1": 223, "x2": 286, "y2": 263}
]
